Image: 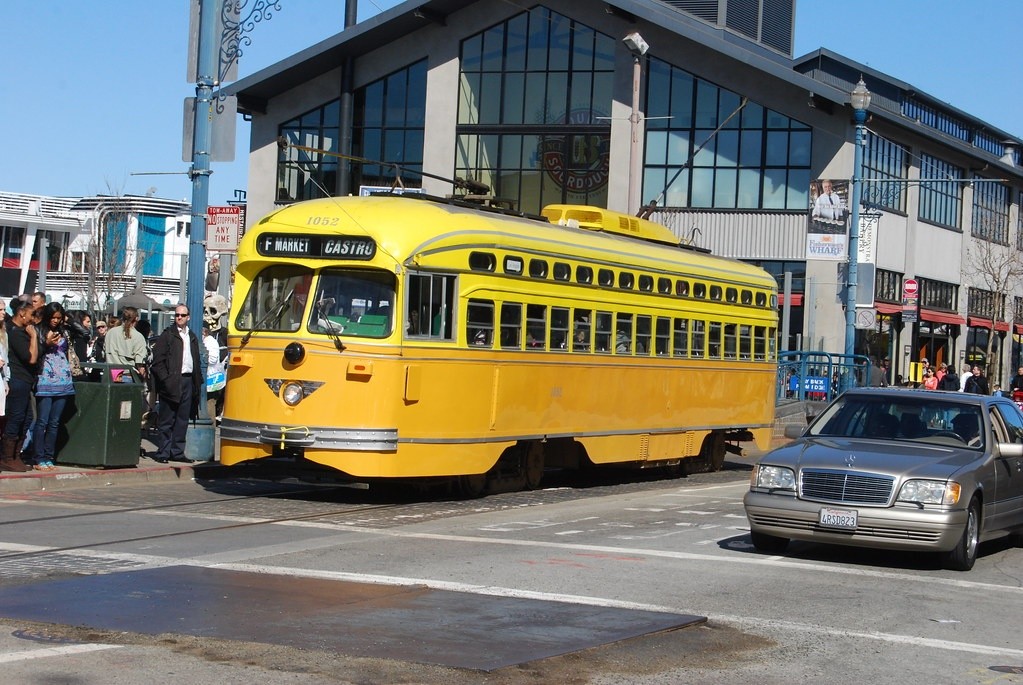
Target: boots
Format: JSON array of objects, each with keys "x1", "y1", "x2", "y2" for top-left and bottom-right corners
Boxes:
[{"x1": 0, "y1": 433, "x2": 27, "y2": 471}]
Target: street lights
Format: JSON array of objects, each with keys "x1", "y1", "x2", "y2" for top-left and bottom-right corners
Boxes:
[
  {"x1": 623, "y1": 31, "x2": 649, "y2": 216},
  {"x1": 844, "y1": 74, "x2": 871, "y2": 393}
]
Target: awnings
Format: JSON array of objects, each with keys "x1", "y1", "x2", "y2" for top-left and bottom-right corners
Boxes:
[
  {"x1": 874, "y1": 302, "x2": 966, "y2": 324},
  {"x1": 968, "y1": 317, "x2": 1009, "y2": 332},
  {"x1": 1013, "y1": 323, "x2": 1023, "y2": 334}
]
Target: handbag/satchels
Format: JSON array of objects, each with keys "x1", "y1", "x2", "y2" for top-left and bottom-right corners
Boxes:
[
  {"x1": 64, "y1": 330, "x2": 81, "y2": 377},
  {"x1": 206, "y1": 362, "x2": 227, "y2": 392}
]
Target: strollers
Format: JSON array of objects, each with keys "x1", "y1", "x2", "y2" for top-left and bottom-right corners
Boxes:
[{"x1": 1011, "y1": 391, "x2": 1023, "y2": 411}]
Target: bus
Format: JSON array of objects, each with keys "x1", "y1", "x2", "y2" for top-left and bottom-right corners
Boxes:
[{"x1": 217, "y1": 192, "x2": 777, "y2": 499}]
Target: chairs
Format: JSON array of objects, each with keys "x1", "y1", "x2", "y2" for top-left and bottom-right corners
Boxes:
[{"x1": 898, "y1": 412, "x2": 925, "y2": 438}]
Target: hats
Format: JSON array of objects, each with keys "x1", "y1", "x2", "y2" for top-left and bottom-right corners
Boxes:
[{"x1": 96, "y1": 321, "x2": 106, "y2": 328}]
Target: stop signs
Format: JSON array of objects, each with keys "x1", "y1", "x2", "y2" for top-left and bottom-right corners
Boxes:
[{"x1": 904, "y1": 279, "x2": 918, "y2": 294}]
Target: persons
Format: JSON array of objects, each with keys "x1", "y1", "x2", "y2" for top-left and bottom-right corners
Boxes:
[
  {"x1": 781, "y1": 355, "x2": 1023, "y2": 404},
  {"x1": 0, "y1": 291, "x2": 229, "y2": 472},
  {"x1": 298, "y1": 284, "x2": 645, "y2": 354},
  {"x1": 811, "y1": 180, "x2": 839, "y2": 227}
]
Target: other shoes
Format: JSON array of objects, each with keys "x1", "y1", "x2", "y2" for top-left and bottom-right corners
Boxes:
[
  {"x1": 159, "y1": 457, "x2": 168, "y2": 463},
  {"x1": 179, "y1": 456, "x2": 194, "y2": 463},
  {"x1": 34, "y1": 465, "x2": 58, "y2": 472}
]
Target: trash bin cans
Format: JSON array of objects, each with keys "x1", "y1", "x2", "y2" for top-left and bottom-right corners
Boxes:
[{"x1": 54, "y1": 362, "x2": 145, "y2": 469}]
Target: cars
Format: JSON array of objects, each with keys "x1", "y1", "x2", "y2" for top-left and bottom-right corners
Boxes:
[{"x1": 743, "y1": 386, "x2": 1023, "y2": 571}]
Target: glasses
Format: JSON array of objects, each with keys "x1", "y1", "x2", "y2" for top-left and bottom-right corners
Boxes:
[
  {"x1": 926, "y1": 370, "x2": 931, "y2": 373},
  {"x1": 97, "y1": 326, "x2": 104, "y2": 329},
  {"x1": 175, "y1": 314, "x2": 189, "y2": 317}
]
